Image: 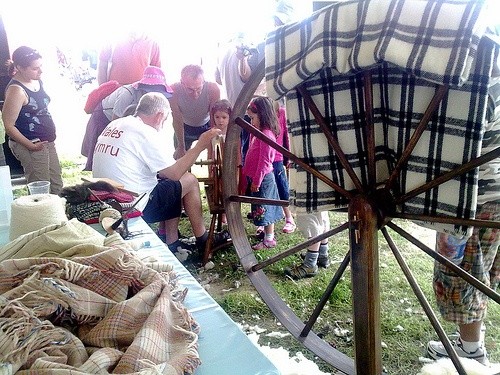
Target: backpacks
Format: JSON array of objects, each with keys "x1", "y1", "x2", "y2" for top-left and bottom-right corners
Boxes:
[{"x1": 84, "y1": 80, "x2": 133, "y2": 114}]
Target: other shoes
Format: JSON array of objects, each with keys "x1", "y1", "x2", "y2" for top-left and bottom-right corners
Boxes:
[
  {"x1": 282, "y1": 219, "x2": 297, "y2": 234},
  {"x1": 157, "y1": 230, "x2": 189, "y2": 243},
  {"x1": 196, "y1": 229, "x2": 230, "y2": 253},
  {"x1": 169, "y1": 242, "x2": 198, "y2": 261},
  {"x1": 251, "y1": 232, "x2": 277, "y2": 249}
]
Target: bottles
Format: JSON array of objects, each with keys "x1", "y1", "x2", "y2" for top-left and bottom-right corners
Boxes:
[{"x1": 447, "y1": 234, "x2": 467, "y2": 264}]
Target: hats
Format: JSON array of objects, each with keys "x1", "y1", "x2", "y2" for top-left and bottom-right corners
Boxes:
[{"x1": 133, "y1": 66, "x2": 174, "y2": 94}]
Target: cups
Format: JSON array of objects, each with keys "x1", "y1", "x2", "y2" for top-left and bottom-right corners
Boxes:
[{"x1": 27, "y1": 181, "x2": 51, "y2": 194}]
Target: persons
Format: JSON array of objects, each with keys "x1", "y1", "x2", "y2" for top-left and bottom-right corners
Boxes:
[
  {"x1": 236, "y1": 1, "x2": 297, "y2": 85},
  {"x1": 427, "y1": 22, "x2": 500, "y2": 364},
  {"x1": 0, "y1": 13, "x2": 25, "y2": 175},
  {"x1": 3, "y1": 45, "x2": 64, "y2": 195},
  {"x1": 98, "y1": 30, "x2": 162, "y2": 85},
  {"x1": 216, "y1": 29, "x2": 265, "y2": 184},
  {"x1": 206, "y1": 99, "x2": 242, "y2": 224},
  {"x1": 93, "y1": 90, "x2": 233, "y2": 265},
  {"x1": 283, "y1": 210, "x2": 331, "y2": 280},
  {"x1": 240, "y1": 96, "x2": 284, "y2": 249},
  {"x1": 167, "y1": 63, "x2": 222, "y2": 217},
  {"x1": 81, "y1": 66, "x2": 173, "y2": 170}
]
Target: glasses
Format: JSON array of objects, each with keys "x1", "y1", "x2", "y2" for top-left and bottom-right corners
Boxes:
[{"x1": 25, "y1": 48, "x2": 39, "y2": 58}]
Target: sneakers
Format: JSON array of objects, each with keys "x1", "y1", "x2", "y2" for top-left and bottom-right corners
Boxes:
[
  {"x1": 300, "y1": 252, "x2": 331, "y2": 268},
  {"x1": 447, "y1": 326, "x2": 485, "y2": 339},
  {"x1": 285, "y1": 262, "x2": 318, "y2": 279},
  {"x1": 427, "y1": 337, "x2": 484, "y2": 364}
]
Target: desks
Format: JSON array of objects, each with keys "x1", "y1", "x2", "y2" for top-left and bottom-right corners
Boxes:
[{"x1": 0, "y1": 216, "x2": 279, "y2": 375}]
